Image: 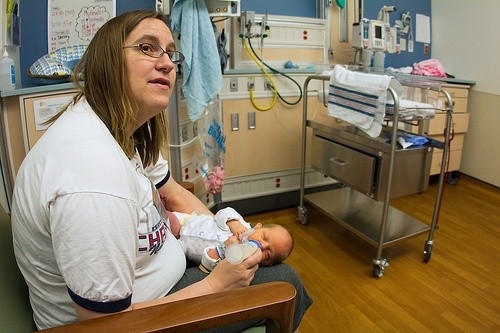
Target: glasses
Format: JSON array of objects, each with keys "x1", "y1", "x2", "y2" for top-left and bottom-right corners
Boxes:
[{"x1": 122, "y1": 43, "x2": 186, "y2": 65}]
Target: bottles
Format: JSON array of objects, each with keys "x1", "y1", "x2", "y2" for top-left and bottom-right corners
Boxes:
[
  {"x1": 0, "y1": 48, "x2": 15, "y2": 93},
  {"x1": 225, "y1": 240, "x2": 262, "y2": 266}
]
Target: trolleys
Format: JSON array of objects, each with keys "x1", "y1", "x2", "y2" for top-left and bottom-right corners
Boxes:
[{"x1": 297, "y1": 71, "x2": 454, "y2": 278}]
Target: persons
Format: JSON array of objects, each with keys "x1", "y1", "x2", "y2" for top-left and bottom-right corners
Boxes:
[
  {"x1": 159, "y1": 193, "x2": 294, "y2": 266},
  {"x1": 11, "y1": 10, "x2": 313, "y2": 333}
]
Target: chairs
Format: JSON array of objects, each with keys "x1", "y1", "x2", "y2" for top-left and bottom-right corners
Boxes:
[{"x1": 0, "y1": 202, "x2": 297, "y2": 333}]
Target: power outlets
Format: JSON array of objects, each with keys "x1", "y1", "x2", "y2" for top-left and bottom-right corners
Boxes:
[
  {"x1": 247, "y1": 76, "x2": 256, "y2": 91},
  {"x1": 231, "y1": 78, "x2": 238, "y2": 93},
  {"x1": 264, "y1": 77, "x2": 271, "y2": 91}
]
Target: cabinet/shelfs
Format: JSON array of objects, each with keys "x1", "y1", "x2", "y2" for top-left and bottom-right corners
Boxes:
[
  {"x1": 3, "y1": 88, "x2": 80, "y2": 201},
  {"x1": 429, "y1": 83, "x2": 471, "y2": 177}
]
